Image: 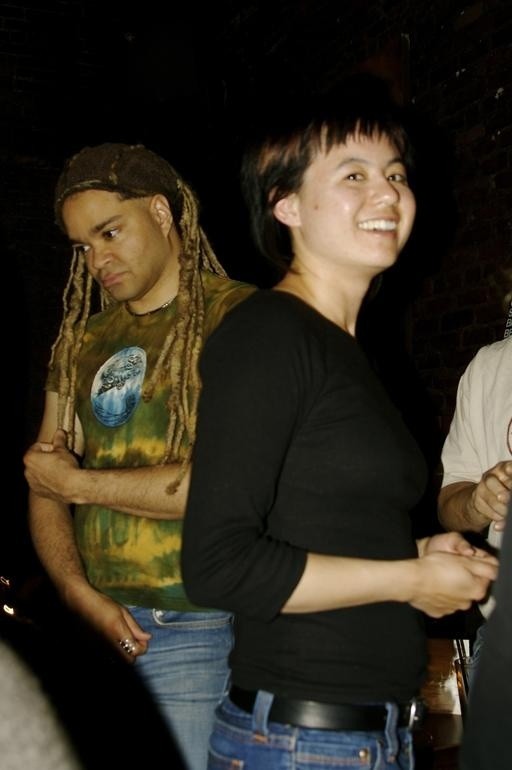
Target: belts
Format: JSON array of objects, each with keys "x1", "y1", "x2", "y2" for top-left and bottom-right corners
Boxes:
[{"x1": 228, "y1": 685, "x2": 418, "y2": 732}]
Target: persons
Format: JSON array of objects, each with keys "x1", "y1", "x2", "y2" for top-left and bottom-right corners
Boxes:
[
  {"x1": 173, "y1": 92, "x2": 499, "y2": 770},
  {"x1": 17, "y1": 141, "x2": 262, "y2": 770},
  {"x1": 430, "y1": 300, "x2": 512, "y2": 654}
]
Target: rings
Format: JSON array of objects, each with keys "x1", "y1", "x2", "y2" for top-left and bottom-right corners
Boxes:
[{"x1": 119, "y1": 635, "x2": 136, "y2": 657}]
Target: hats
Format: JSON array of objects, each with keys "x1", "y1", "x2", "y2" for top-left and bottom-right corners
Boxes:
[{"x1": 55, "y1": 143, "x2": 183, "y2": 232}]
